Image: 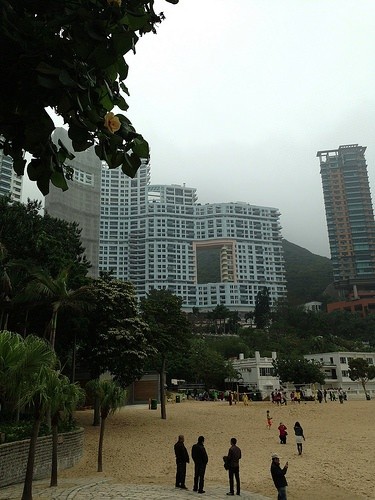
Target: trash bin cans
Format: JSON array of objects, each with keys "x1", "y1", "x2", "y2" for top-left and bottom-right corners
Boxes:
[
  {"x1": 176, "y1": 395, "x2": 180, "y2": 403},
  {"x1": 151, "y1": 399, "x2": 157, "y2": 410}
]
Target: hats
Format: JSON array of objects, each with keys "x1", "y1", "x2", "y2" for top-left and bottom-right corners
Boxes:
[{"x1": 272, "y1": 453, "x2": 282, "y2": 459}]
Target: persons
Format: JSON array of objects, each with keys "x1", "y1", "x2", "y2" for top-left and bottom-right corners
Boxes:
[
  {"x1": 223, "y1": 438, "x2": 242, "y2": 496},
  {"x1": 271, "y1": 389, "x2": 348, "y2": 406},
  {"x1": 174, "y1": 435, "x2": 189, "y2": 489},
  {"x1": 195, "y1": 390, "x2": 248, "y2": 406},
  {"x1": 266, "y1": 410, "x2": 273, "y2": 430},
  {"x1": 192, "y1": 436, "x2": 208, "y2": 493},
  {"x1": 278, "y1": 422, "x2": 287, "y2": 444},
  {"x1": 271, "y1": 453, "x2": 288, "y2": 500},
  {"x1": 293, "y1": 421, "x2": 305, "y2": 455}
]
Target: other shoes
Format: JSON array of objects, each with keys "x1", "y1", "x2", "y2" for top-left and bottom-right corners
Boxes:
[
  {"x1": 193, "y1": 489, "x2": 197, "y2": 491},
  {"x1": 198, "y1": 490, "x2": 205, "y2": 493},
  {"x1": 227, "y1": 493, "x2": 234, "y2": 495},
  {"x1": 181, "y1": 486, "x2": 188, "y2": 489},
  {"x1": 176, "y1": 485, "x2": 181, "y2": 487},
  {"x1": 236, "y1": 493, "x2": 240, "y2": 495}
]
